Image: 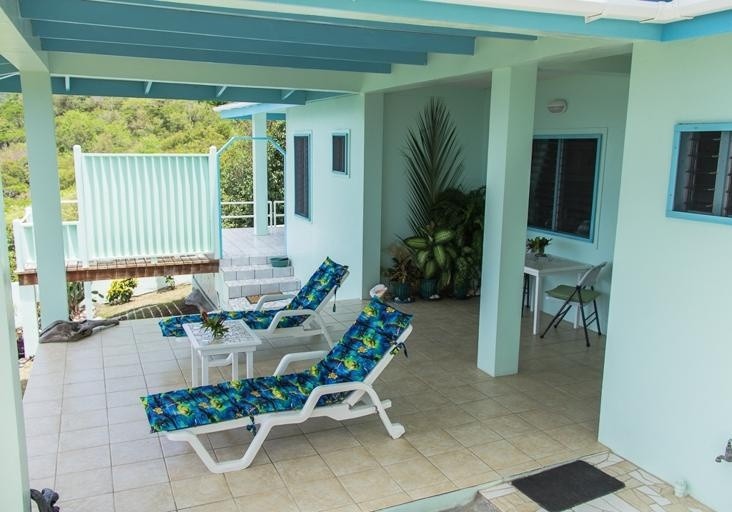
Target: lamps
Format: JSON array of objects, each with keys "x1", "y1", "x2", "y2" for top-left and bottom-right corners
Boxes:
[{"x1": 546, "y1": 99, "x2": 568, "y2": 115}]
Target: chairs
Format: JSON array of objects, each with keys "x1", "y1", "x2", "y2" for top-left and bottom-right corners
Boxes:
[{"x1": 540, "y1": 262, "x2": 606, "y2": 348}]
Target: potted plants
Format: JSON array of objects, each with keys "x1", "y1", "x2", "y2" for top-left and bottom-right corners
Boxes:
[
  {"x1": 526, "y1": 236, "x2": 553, "y2": 262},
  {"x1": 379, "y1": 95, "x2": 486, "y2": 304}
]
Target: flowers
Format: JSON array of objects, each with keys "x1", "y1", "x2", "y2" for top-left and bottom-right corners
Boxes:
[{"x1": 106, "y1": 277, "x2": 136, "y2": 303}]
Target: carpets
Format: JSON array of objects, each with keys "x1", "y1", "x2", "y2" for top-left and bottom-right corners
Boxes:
[{"x1": 511, "y1": 461, "x2": 625, "y2": 512}]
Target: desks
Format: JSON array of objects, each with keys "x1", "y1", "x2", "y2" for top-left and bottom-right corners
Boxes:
[{"x1": 524, "y1": 253, "x2": 594, "y2": 337}]
